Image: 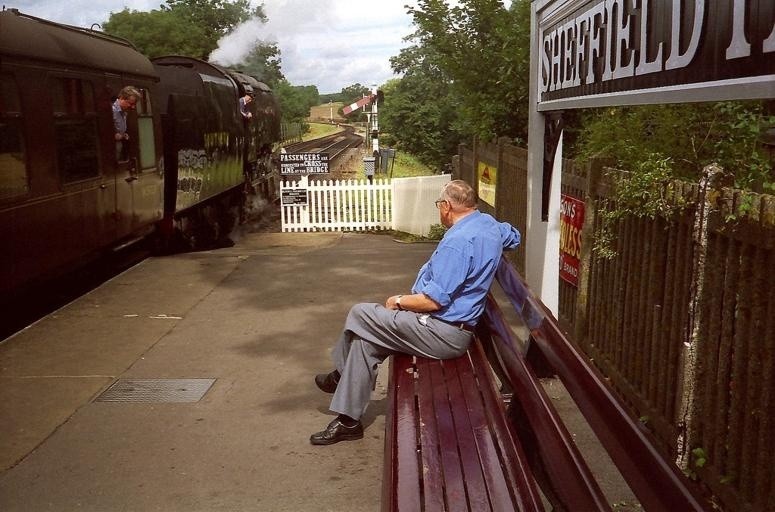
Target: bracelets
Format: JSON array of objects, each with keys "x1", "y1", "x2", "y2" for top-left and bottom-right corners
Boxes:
[{"x1": 394, "y1": 294, "x2": 404, "y2": 311}]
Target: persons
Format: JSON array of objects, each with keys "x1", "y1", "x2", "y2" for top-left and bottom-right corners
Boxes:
[
  {"x1": 237, "y1": 93, "x2": 255, "y2": 118},
  {"x1": 307, "y1": 178, "x2": 524, "y2": 447},
  {"x1": 111, "y1": 84, "x2": 145, "y2": 162}
]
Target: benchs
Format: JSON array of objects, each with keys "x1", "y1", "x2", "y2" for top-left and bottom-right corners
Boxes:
[{"x1": 379, "y1": 254, "x2": 716, "y2": 511}]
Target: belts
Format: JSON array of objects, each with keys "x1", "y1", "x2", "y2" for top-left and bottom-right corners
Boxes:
[{"x1": 454, "y1": 322, "x2": 470, "y2": 330}]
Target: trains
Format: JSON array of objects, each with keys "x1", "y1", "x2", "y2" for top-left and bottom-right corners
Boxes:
[{"x1": 0, "y1": 3, "x2": 285, "y2": 341}]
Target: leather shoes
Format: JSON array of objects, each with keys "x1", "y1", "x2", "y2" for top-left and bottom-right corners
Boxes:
[
  {"x1": 310, "y1": 419, "x2": 363, "y2": 445},
  {"x1": 315, "y1": 370, "x2": 341, "y2": 393}
]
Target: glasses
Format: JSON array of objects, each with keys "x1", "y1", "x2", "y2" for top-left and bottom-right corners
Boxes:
[{"x1": 435, "y1": 199, "x2": 447, "y2": 209}]
